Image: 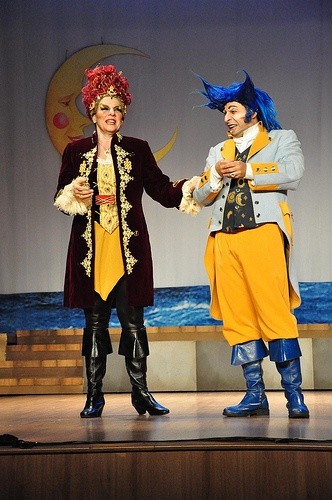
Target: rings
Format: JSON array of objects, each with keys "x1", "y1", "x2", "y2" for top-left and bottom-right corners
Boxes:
[
  {"x1": 230, "y1": 173, "x2": 235, "y2": 177},
  {"x1": 80, "y1": 194, "x2": 82, "y2": 199}
]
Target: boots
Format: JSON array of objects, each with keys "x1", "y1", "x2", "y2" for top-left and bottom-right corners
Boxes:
[
  {"x1": 268, "y1": 338, "x2": 310, "y2": 418},
  {"x1": 79, "y1": 329, "x2": 113, "y2": 417},
  {"x1": 222, "y1": 338, "x2": 269, "y2": 416},
  {"x1": 118, "y1": 327, "x2": 169, "y2": 415}
]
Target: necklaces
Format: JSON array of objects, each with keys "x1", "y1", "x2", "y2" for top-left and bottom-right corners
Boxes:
[{"x1": 96, "y1": 140, "x2": 111, "y2": 155}]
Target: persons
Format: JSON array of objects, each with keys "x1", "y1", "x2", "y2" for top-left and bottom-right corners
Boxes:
[
  {"x1": 192, "y1": 70, "x2": 311, "y2": 419},
  {"x1": 53, "y1": 66, "x2": 203, "y2": 419}
]
